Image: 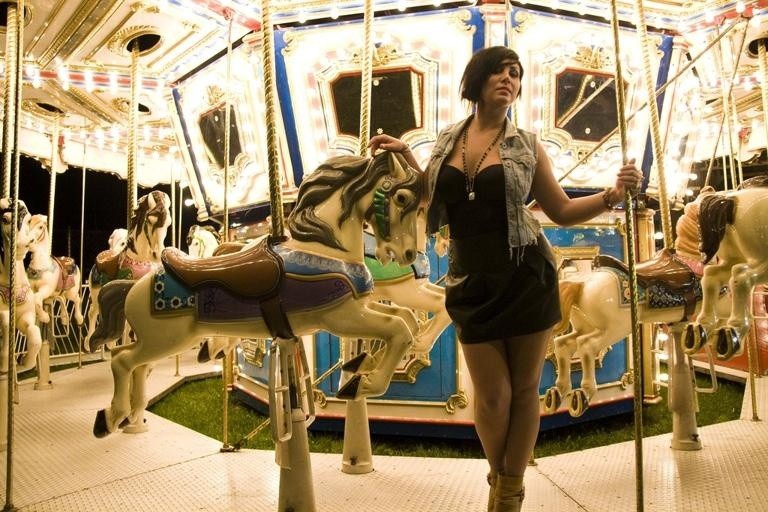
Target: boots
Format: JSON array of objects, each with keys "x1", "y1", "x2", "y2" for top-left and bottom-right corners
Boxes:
[
  {"x1": 486, "y1": 473, "x2": 497, "y2": 511},
  {"x1": 493, "y1": 473, "x2": 526, "y2": 512}
]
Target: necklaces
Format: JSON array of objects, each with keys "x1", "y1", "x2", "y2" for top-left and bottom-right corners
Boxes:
[{"x1": 459, "y1": 123, "x2": 505, "y2": 201}]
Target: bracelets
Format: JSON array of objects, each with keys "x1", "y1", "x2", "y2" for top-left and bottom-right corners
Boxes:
[
  {"x1": 602, "y1": 184, "x2": 625, "y2": 213},
  {"x1": 400, "y1": 144, "x2": 411, "y2": 154}
]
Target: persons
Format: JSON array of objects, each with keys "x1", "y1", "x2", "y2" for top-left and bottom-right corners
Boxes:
[{"x1": 364, "y1": 43, "x2": 641, "y2": 512}]
